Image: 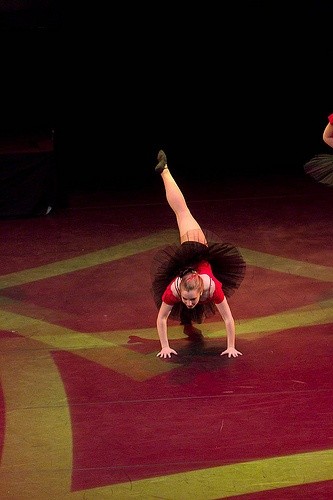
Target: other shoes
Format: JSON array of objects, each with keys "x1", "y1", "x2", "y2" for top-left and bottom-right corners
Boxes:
[{"x1": 155, "y1": 150, "x2": 169, "y2": 175}]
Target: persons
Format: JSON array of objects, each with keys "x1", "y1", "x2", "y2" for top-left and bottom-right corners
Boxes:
[
  {"x1": 148, "y1": 150, "x2": 246, "y2": 359},
  {"x1": 322, "y1": 114, "x2": 333, "y2": 147}
]
